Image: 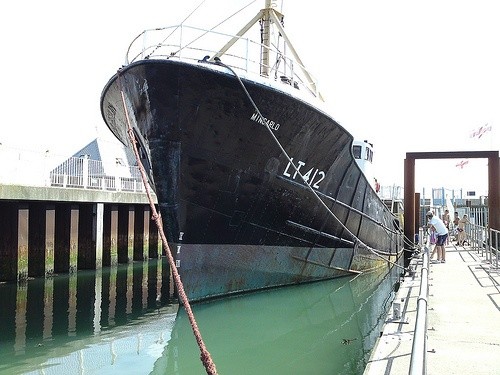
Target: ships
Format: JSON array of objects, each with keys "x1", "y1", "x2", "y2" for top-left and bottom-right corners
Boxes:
[{"x1": 98, "y1": 0, "x2": 406, "y2": 305}]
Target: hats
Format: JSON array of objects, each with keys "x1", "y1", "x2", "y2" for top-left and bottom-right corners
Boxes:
[{"x1": 425, "y1": 211, "x2": 433, "y2": 217}]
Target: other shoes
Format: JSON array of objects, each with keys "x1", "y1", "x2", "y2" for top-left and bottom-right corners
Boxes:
[
  {"x1": 432, "y1": 260, "x2": 441, "y2": 264},
  {"x1": 441, "y1": 260, "x2": 446, "y2": 263}
]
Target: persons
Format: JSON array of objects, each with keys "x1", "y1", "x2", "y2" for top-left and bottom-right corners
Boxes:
[
  {"x1": 442, "y1": 209, "x2": 450, "y2": 246},
  {"x1": 375, "y1": 179, "x2": 380, "y2": 195},
  {"x1": 457, "y1": 214, "x2": 475, "y2": 246},
  {"x1": 452, "y1": 211, "x2": 460, "y2": 241},
  {"x1": 425, "y1": 211, "x2": 449, "y2": 263}
]
